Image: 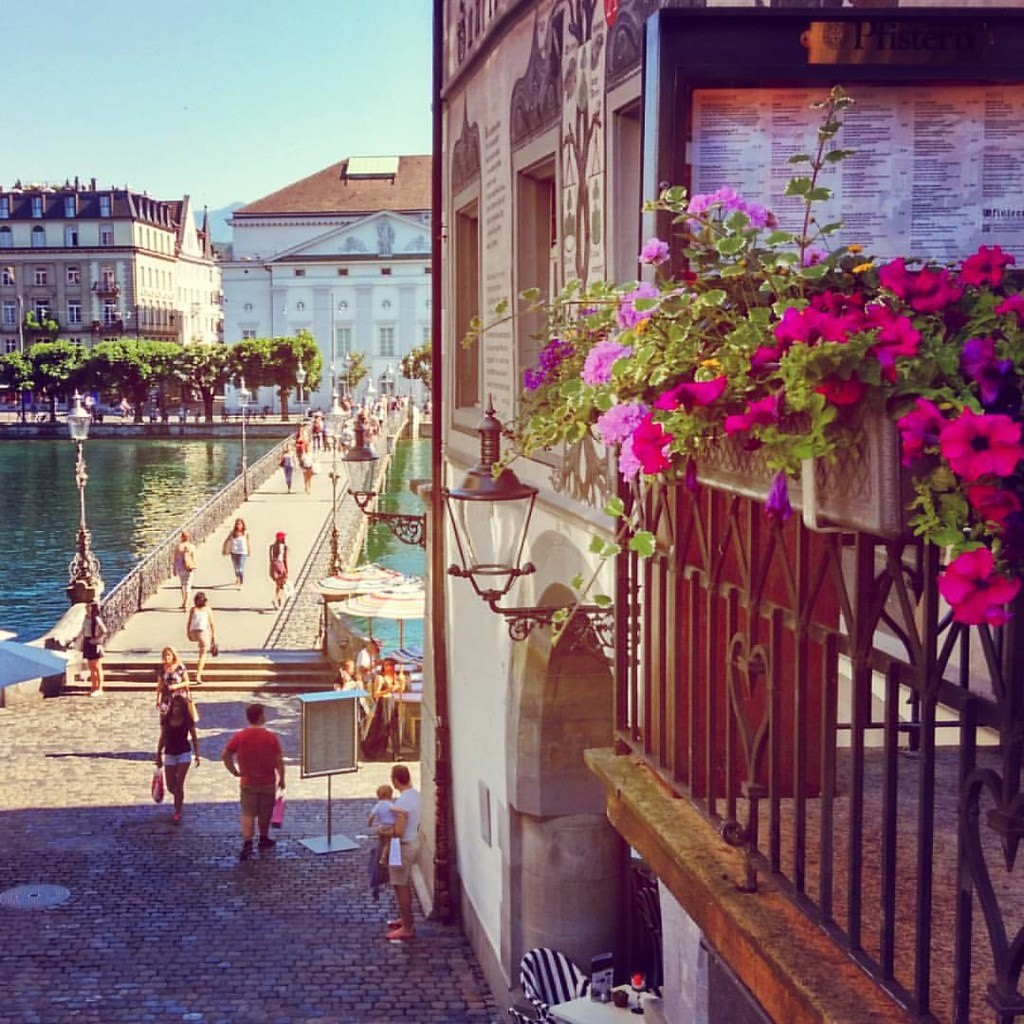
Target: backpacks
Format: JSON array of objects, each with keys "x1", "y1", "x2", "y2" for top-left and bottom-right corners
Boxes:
[{"x1": 368, "y1": 848, "x2": 390, "y2": 900}]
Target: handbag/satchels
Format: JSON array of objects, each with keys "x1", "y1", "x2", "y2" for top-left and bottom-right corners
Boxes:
[
  {"x1": 223, "y1": 534, "x2": 232, "y2": 555},
  {"x1": 387, "y1": 830, "x2": 404, "y2": 866},
  {"x1": 183, "y1": 547, "x2": 198, "y2": 569},
  {"x1": 210, "y1": 640, "x2": 218, "y2": 656},
  {"x1": 188, "y1": 697, "x2": 199, "y2": 723},
  {"x1": 271, "y1": 784, "x2": 283, "y2": 828},
  {"x1": 151, "y1": 765, "x2": 164, "y2": 804}
]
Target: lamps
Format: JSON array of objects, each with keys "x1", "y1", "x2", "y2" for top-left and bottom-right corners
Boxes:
[
  {"x1": 438, "y1": 394, "x2": 642, "y2": 666},
  {"x1": 341, "y1": 424, "x2": 427, "y2": 549}
]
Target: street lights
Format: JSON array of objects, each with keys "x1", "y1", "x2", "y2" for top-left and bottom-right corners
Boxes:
[
  {"x1": 321, "y1": 434, "x2": 344, "y2": 577},
  {"x1": 64, "y1": 387, "x2": 104, "y2": 603},
  {"x1": 236, "y1": 379, "x2": 252, "y2": 500},
  {"x1": 407, "y1": 352, "x2": 415, "y2": 403},
  {"x1": 293, "y1": 362, "x2": 306, "y2": 423}
]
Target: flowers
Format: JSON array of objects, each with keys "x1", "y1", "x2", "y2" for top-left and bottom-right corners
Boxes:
[{"x1": 462, "y1": 84, "x2": 1024, "y2": 645}]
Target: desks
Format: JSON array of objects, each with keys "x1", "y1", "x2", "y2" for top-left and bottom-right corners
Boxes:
[
  {"x1": 381, "y1": 692, "x2": 421, "y2": 753},
  {"x1": 548, "y1": 984, "x2": 658, "y2": 1024},
  {"x1": 375, "y1": 664, "x2": 417, "y2": 673}
]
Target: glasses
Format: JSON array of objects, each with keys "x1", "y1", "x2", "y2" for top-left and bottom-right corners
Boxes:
[{"x1": 85, "y1": 605, "x2": 91, "y2": 608}]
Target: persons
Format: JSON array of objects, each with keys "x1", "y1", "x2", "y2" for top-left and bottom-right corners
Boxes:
[
  {"x1": 222, "y1": 703, "x2": 284, "y2": 858},
  {"x1": 293, "y1": 393, "x2": 432, "y2": 468},
  {"x1": 82, "y1": 602, "x2": 107, "y2": 696},
  {"x1": 367, "y1": 785, "x2": 407, "y2": 865},
  {"x1": 188, "y1": 592, "x2": 215, "y2": 683},
  {"x1": 269, "y1": 532, "x2": 288, "y2": 610},
  {"x1": 301, "y1": 443, "x2": 313, "y2": 492},
  {"x1": 223, "y1": 519, "x2": 251, "y2": 590},
  {"x1": 86, "y1": 406, "x2": 270, "y2": 426},
  {"x1": 156, "y1": 695, "x2": 201, "y2": 822},
  {"x1": 333, "y1": 638, "x2": 404, "y2": 762},
  {"x1": 174, "y1": 532, "x2": 196, "y2": 611},
  {"x1": 383, "y1": 766, "x2": 421, "y2": 941},
  {"x1": 283, "y1": 443, "x2": 297, "y2": 493}
]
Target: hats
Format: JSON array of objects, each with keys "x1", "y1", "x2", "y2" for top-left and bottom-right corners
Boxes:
[
  {"x1": 276, "y1": 532, "x2": 286, "y2": 539},
  {"x1": 371, "y1": 637, "x2": 382, "y2": 649}
]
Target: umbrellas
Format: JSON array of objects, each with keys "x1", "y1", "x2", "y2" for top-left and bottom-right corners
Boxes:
[
  {"x1": 316, "y1": 563, "x2": 402, "y2": 671},
  {"x1": 325, "y1": 577, "x2": 427, "y2": 703},
  {"x1": 0, "y1": 641, "x2": 68, "y2": 688}
]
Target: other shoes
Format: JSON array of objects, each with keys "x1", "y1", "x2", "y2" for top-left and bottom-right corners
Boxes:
[
  {"x1": 94, "y1": 643, "x2": 104, "y2": 654},
  {"x1": 387, "y1": 917, "x2": 415, "y2": 926},
  {"x1": 196, "y1": 674, "x2": 201, "y2": 684},
  {"x1": 87, "y1": 689, "x2": 103, "y2": 697},
  {"x1": 182, "y1": 600, "x2": 187, "y2": 612},
  {"x1": 258, "y1": 835, "x2": 276, "y2": 846},
  {"x1": 240, "y1": 839, "x2": 254, "y2": 860},
  {"x1": 273, "y1": 598, "x2": 281, "y2": 610},
  {"x1": 173, "y1": 810, "x2": 181, "y2": 823},
  {"x1": 386, "y1": 928, "x2": 413, "y2": 938}
]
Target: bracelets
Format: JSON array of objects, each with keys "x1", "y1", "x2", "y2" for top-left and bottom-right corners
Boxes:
[{"x1": 157, "y1": 752, "x2": 162, "y2": 756}]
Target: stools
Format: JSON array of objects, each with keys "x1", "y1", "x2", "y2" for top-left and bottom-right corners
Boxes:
[{"x1": 405, "y1": 714, "x2": 421, "y2": 746}]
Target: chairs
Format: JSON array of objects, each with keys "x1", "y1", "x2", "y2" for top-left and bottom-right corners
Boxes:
[{"x1": 520, "y1": 948, "x2": 591, "y2": 1024}]
